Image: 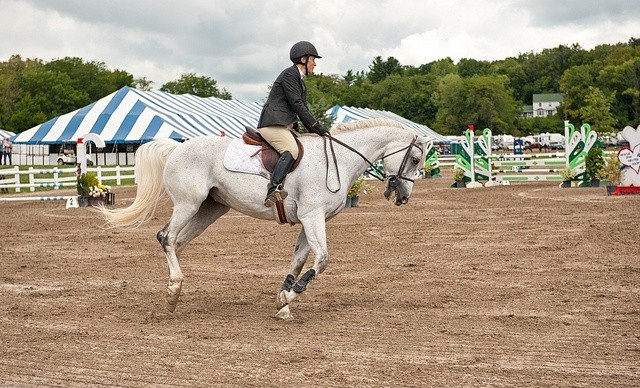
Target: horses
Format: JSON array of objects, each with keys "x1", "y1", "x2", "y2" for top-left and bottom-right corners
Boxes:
[
  {"x1": 86, "y1": 117, "x2": 436, "y2": 322},
  {"x1": 525, "y1": 140, "x2": 542, "y2": 152}
]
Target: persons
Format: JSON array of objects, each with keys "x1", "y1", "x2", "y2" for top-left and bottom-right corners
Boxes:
[
  {"x1": 3, "y1": 137, "x2": 13, "y2": 165},
  {"x1": 256, "y1": 41, "x2": 326, "y2": 207},
  {"x1": 0, "y1": 139, "x2": 3, "y2": 165}
]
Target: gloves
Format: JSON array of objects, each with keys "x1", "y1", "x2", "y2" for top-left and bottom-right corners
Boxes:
[{"x1": 314, "y1": 121, "x2": 326, "y2": 134}]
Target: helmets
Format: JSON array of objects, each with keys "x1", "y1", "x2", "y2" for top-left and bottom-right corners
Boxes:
[{"x1": 290, "y1": 41, "x2": 322, "y2": 59}]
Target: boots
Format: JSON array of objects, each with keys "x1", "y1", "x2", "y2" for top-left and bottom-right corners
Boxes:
[{"x1": 264, "y1": 151, "x2": 295, "y2": 208}]
